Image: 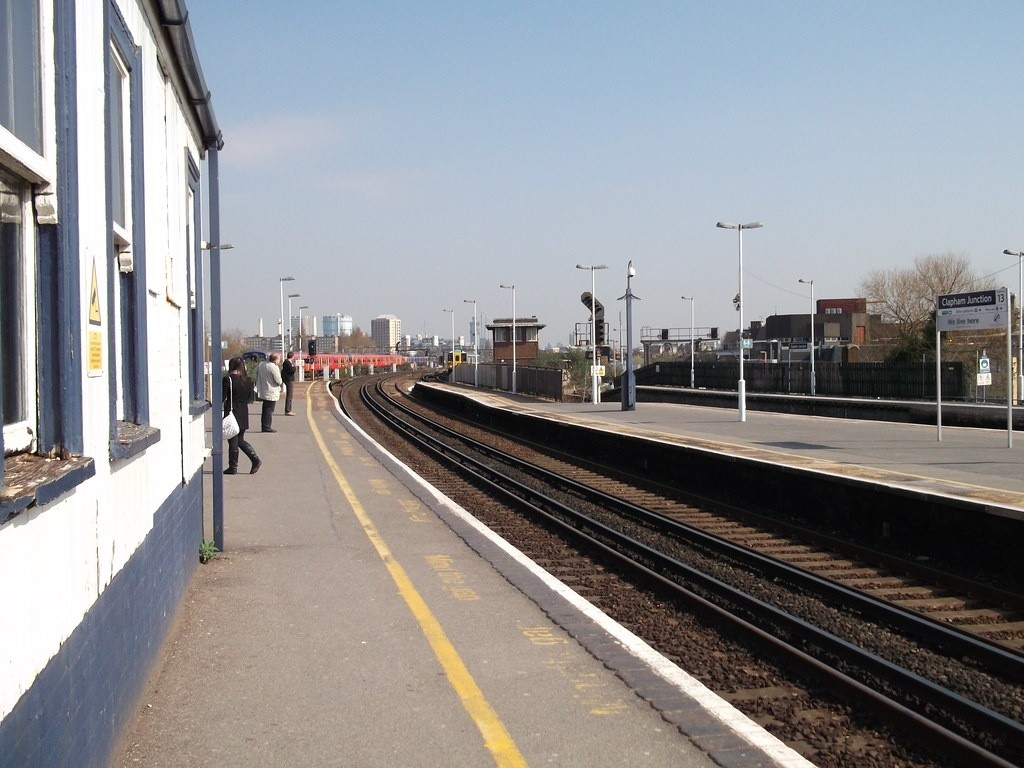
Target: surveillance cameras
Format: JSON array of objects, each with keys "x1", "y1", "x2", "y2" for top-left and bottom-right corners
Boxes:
[{"x1": 629, "y1": 269, "x2": 635, "y2": 277}]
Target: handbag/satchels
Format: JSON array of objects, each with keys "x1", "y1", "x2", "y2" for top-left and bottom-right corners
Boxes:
[{"x1": 221, "y1": 412, "x2": 239, "y2": 440}]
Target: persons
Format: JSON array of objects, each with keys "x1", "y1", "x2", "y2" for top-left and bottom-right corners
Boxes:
[
  {"x1": 222, "y1": 357, "x2": 261, "y2": 475},
  {"x1": 257, "y1": 353, "x2": 282, "y2": 432},
  {"x1": 280, "y1": 352, "x2": 297, "y2": 416}
]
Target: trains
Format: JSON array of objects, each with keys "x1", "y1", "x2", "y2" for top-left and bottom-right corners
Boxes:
[{"x1": 448, "y1": 349, "x2": 480, "y2": 368}]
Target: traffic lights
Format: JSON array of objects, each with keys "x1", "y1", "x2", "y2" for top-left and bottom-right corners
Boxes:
[
  {"x1": 308, "y1": 340, "x2": 316, "y2": 355},
  {"x1": 595, "y1": 317, "x2": 604, "y2": 345},
  {"x1": 581, "y1": 291, "x2": 604, "y2": 317}
]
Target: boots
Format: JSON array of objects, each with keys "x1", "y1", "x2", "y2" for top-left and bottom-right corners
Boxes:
[
  {"x1": 242, "y1": 441, "x2": 262, "y2": 474},
  {"x1": 223, "y1": 449, "x2": 239, "y2": 474}
]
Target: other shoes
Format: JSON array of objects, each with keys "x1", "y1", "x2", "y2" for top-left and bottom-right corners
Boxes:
[
  {"x1": 262, "y1": 428, "x2": 276, "y2": 432},
  {"x1": 285, "y1": 412, "x2": 296, "y2": 416}
]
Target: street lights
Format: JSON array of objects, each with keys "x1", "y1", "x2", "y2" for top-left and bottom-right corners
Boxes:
[
  {"x1": 1003, "y1": 250, "x2": 1024, "y2": 404},
  {"x1": 715, "y1": 221, "x2": 763, "y2": 422},
  {"x1": 574, "y1": 264, "x2": 609, "y2": 404},
  {"x1": 682, "y1": 296, "x2": 695, "y2": 389},
  {"x1": 500, "y1": 284, "x2": 516, "y2": 392},
  {"x1": 288, "y1": 294, "x2": 300, "y2": 352},
  {"x1": 799, "y1": 279, "x2": 816, "y2": 396},
  {"x1": 443, "y1": 309, "x2": 455, "y2": 383},
  {"x1": 464, "y1": 300, "x2": 478, "y2": 388},
  {"x1": 299, "y1": 306, "x2": 308, "y2": 382},
  {"x1": 280, "y1": 276, "x2": 295, "y2": 363}
]
